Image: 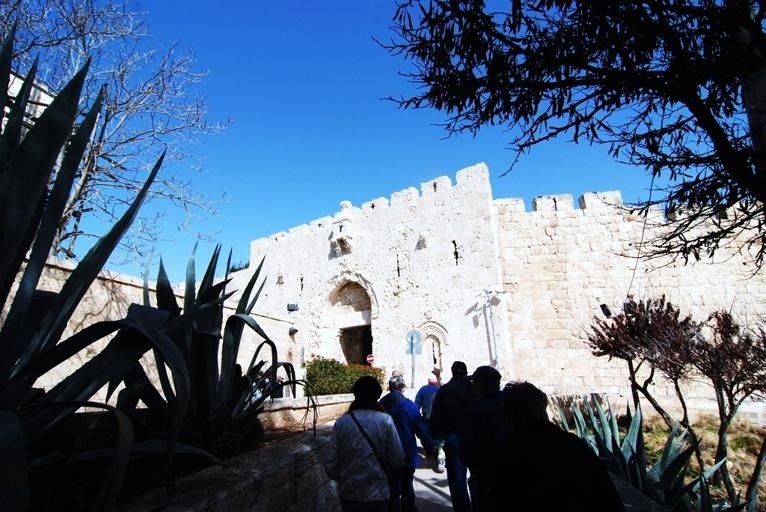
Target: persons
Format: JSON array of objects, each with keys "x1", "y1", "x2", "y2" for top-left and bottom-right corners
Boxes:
[
  {"x1": 415, "y1": 361, "x2": 628, "y2": 511},
  {"x1": 378, "y1": 374, "x2": 438, "y2": 512},
  {"x1": 323, "y1": 374, "x2": 406, "y2": 512}
]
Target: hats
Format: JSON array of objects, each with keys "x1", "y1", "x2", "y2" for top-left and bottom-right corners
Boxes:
[
  {"x1": 389, "y1": 375, "x2": 406, "y2": 392},
  {"x1": 465, "y1": 366, "x2": 502, "y2": 388}
]
